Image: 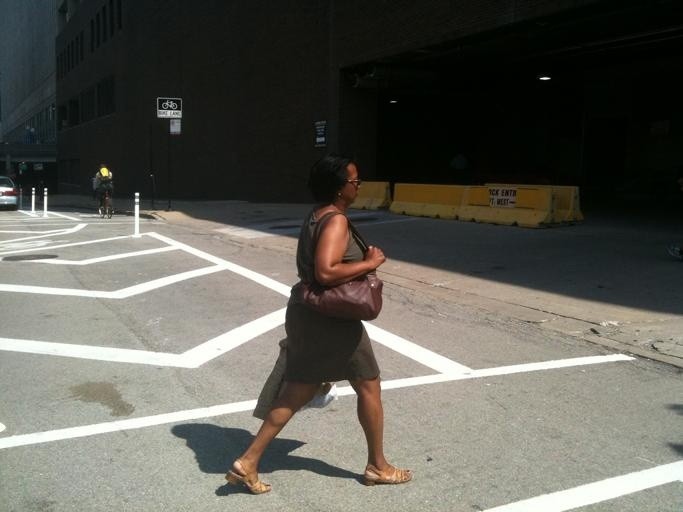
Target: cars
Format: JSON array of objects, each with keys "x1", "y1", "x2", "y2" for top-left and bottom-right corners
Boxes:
[{"x1": 1, "y1": 176, "x2": 17, "y2": 211}]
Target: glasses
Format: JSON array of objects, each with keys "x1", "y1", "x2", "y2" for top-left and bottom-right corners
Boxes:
[{"x1": 344, "y1": 178, "x2": 361, "y2": 185}]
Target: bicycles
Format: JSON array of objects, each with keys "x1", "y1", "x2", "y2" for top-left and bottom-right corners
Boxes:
[{"x1": 98, "y1": 184, "x2": 112, "y2": 219}]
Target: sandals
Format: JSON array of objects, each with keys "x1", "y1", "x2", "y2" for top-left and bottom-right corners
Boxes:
[
  {"x1": 363, "y1": 466, "x2": 412, "y2": 486},
  {"x1": 224, "y1": 458, "x2": 272, "y2": 495}
]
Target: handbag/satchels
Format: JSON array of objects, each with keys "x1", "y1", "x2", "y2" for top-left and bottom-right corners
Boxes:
[
  {"x1": 299, "y1": 212, "x2": 383, "y2": 321},
  {"x1": 253, "y1": 338, "x2": 339, "y2": 421}
]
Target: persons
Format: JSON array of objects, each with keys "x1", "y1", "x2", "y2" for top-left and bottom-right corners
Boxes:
[
  {"x1": 665, "y1": 242, "x2": 682, "y2": 260},
  {"x1": 222, "y1": 152, "x2": 414, "y2": 496},
  {"x1": 92, "y1": 162, "x2": 113, "y2": 211}
]
